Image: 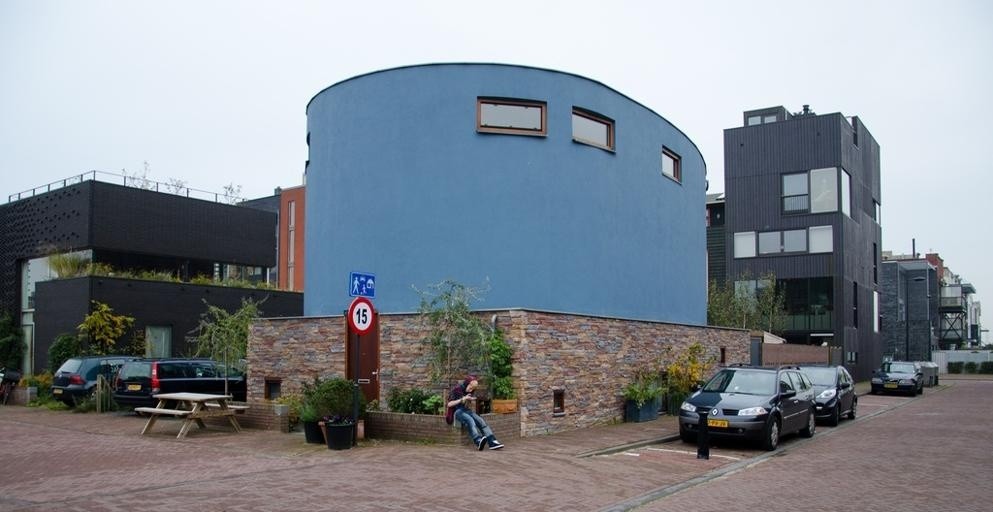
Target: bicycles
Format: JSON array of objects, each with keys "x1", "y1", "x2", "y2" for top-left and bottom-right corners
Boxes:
[{"x1": 0, "y1": 366, "x2": 18, "y2": 406}]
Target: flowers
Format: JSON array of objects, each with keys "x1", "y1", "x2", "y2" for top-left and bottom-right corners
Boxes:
[{"x1": 661, "y1": 342, "x2": 718, "y2": 394}]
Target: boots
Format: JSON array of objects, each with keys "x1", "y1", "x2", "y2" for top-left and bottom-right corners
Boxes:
[
  {"x1": 473, "y1": 435, "x2": 487, "y2": 451},
  {"x1": 487, "y1": 434, "x2": 505, "y2": 450}
]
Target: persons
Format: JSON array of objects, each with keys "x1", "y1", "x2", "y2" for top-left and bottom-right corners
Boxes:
[{"x1": 445, "y1": 374, "x2": 504, "y2": 451}]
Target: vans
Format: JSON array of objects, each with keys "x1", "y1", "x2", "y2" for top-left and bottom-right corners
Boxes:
[{"x1": 51, "y1": 352, "x2": 248, "y2": 415}]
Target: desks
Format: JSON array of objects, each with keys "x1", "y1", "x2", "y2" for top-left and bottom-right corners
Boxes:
[{"x1": 139, "y1": 393, "x2": 242, "y2": 438}]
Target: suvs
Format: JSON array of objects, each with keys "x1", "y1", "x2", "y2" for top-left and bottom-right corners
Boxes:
[{"x1": 679, "y1": 361, "x2": 817, "y2": 450}]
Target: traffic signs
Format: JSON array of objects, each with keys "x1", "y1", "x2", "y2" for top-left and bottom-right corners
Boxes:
[{"x1": 348, "y1": 298, "x2": 376, "y2": 335}]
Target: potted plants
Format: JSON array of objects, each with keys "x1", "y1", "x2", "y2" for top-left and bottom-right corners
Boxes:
[
  {"x1": 621, "y1": 370, "x2": 666, "y2": 422},
  {"x1": 486, "y1": 374, "x2": 517, "y2": 413},
  {"x1": 289, "y1": 376, "x2": 367, "y2": 452}
]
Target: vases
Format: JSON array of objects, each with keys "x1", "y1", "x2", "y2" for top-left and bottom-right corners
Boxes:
[{"x1": 662, "y1": 386, "x2": 688, "y2": 416}]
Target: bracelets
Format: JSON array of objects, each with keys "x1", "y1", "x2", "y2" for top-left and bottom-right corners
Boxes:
[{"x1": 460, "y1": 396, "x2": 465, "y2": 404}]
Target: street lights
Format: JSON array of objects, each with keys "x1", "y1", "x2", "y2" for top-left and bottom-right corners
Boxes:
[{"x1": 904, "y1": 275, "x2": 926, "y2": 362}]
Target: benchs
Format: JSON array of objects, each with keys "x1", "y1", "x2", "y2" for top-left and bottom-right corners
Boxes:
[
  {"x1": 134, "y1": 407, "x2": 195, "y2": 438},
  {"x1": 191, "y1": 403, "x2": 250, "y2": 433}
]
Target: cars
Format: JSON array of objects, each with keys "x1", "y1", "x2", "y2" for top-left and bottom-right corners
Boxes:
[
  {"x1": 869, "y1": 361, "x2": 924, "y2": 396},
  {"x1": 794, "y1": 364, "x2": 859, "y2": 427}
]
